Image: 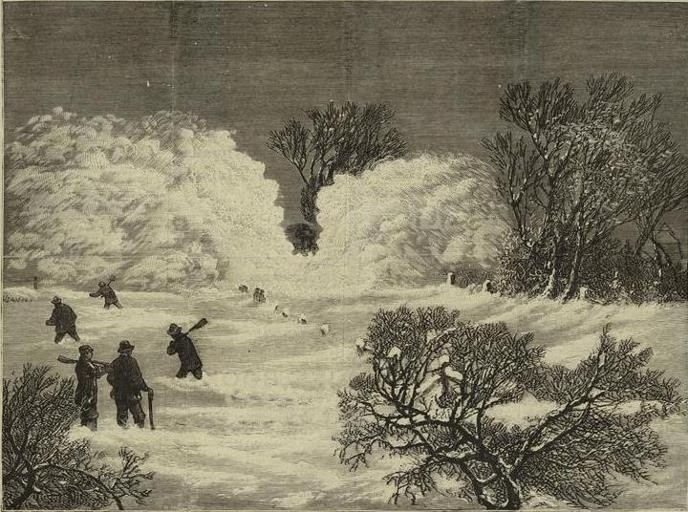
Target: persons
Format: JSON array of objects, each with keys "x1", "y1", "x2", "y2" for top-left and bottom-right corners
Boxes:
[
  {"x1": 45, "y1": 296, "x2": 80, "y2": 344},
  {"x1": 75, "y1": 344, "x2": 107, "y2": 430},
  {"x1": 104, "y1": 340, "x2": 153, "y2": 428},
  {"x1": 89, "y1": 280, "x2": 122, "y2": 310},
  {"x1": 164, "y1": 323, "x2": 206, "y2": 381}
]
